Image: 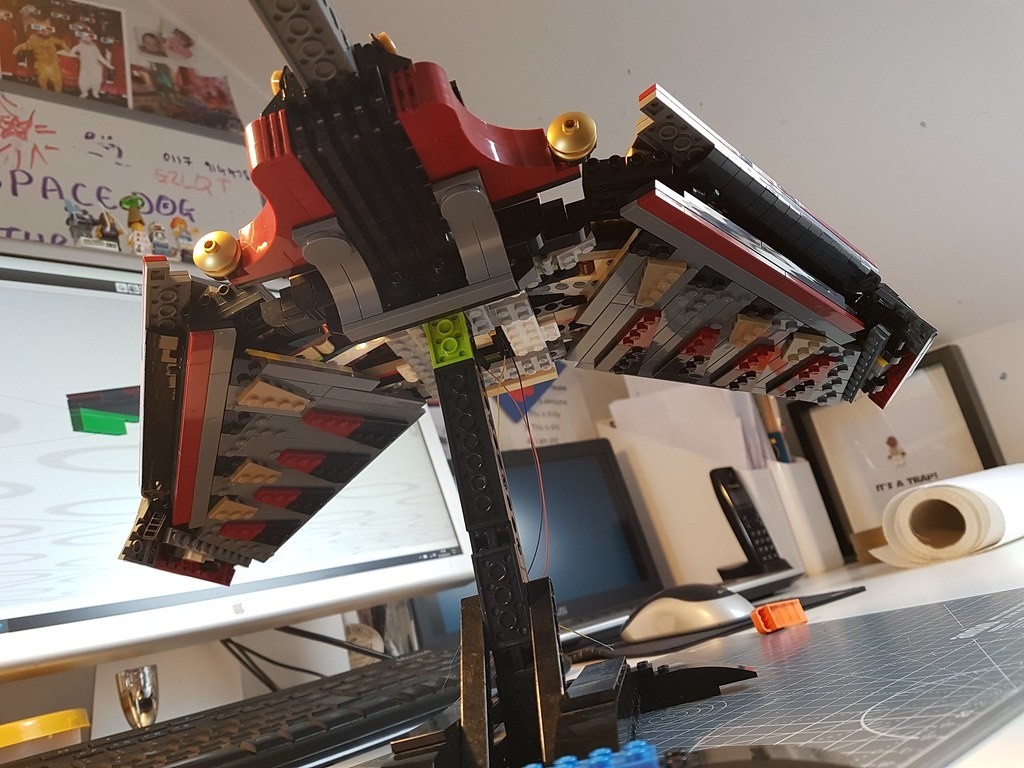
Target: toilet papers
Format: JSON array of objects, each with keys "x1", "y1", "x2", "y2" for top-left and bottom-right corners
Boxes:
[{"x1": 863, "y1": 460, "x2": 1024, "y2": 571}]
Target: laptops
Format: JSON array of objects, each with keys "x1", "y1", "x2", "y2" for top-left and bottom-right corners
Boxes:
[{"x1": 411, "y1": 438, "x2": 804, "y2": 654}]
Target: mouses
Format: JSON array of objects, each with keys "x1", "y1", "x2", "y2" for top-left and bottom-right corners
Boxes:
[{"x1": 620, "y1": 582, "x2": 758, "y2": 645}]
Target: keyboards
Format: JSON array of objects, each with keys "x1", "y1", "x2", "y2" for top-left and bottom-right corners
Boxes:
[{"x1": 1, "y1": 648, "x2": 459, "y2": 768}]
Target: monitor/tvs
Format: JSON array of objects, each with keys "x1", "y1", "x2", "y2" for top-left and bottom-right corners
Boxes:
[{"x1": 1, "y1": 235, "x2": 477, "y2": 677}]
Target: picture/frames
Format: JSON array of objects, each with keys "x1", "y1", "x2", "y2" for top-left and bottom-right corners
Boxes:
[{"x1": 1, "y1": 0, "x2": 265, "y2": 275}]
